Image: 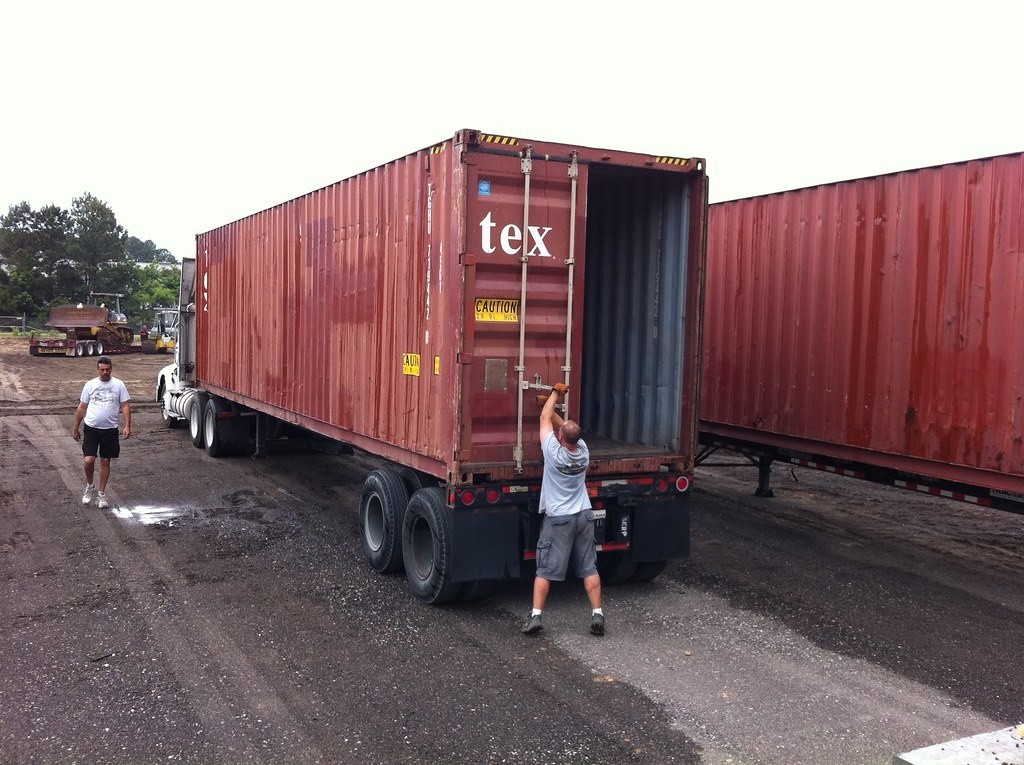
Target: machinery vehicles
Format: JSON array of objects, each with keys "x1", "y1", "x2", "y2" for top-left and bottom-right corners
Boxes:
[
  {"x1": 46, "y1": 291, "x2": 135, "y2": 347},
  {"x1": 141, "y1": 307, "x2": 180, "y2": 354}
]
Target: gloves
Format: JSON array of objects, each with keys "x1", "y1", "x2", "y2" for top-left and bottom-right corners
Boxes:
[
  {"x1": 535, "y1": 395, "x2": 548, "y2": 408},
  {"x1": 551, "y1": 383, "x2": 569, "y2": 397}
]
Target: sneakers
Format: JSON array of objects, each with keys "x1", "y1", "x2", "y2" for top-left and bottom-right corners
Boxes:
[
  {"x1": 520, "y1": 610, "x2": 543, "y2": 635},
  {"x1": 82, "y1": 484, "x2": 96, "y2": 505},
  {"x1": 589, "y1": 612, "x2": 605, "y2": 636},
  {"x1": 94, "y1": 490, "x2": 109, "y2": 509}
]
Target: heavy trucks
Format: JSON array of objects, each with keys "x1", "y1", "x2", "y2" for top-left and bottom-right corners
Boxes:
[{"x1": 156, "y1": 131, "x2": 710, "y2": 608}]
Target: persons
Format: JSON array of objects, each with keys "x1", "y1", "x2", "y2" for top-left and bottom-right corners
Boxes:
[
  {"x1": 521, "y1": 383, "x2": 605, "y2": 636},
  {"x1": 72, "y1": 357, "x2": 131, "y2": 509},
  {"x1": 139, "y1": 325, "x2": 149, "y2": 345}
]
